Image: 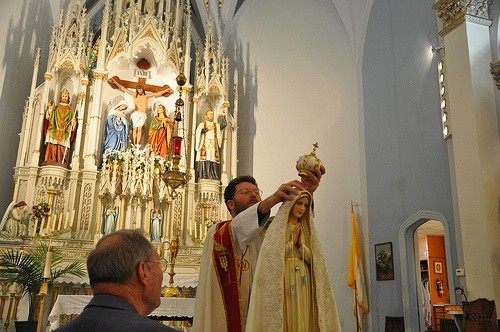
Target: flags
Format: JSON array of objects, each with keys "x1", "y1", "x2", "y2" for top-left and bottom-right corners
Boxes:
[{"x1": 348, "y1": 212, "x2": 369, "y2": 332}]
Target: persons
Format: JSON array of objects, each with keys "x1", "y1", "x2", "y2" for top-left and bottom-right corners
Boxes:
[
  {"x1": 104, "y1": 203, "x2": 116, "y2": 236},
  {"x1": 44, "y1": 87, "x2": 78, "y2": 165},
  {"x1": 148, "y1": 104, "x2": 175, "y2": 162},
  {"x1": 108, "y1": 79, "x2": 172, "y2": 145},
  {"x1": 192, "y1": 165, "x2": 326, "y2": 332},
  {"x1": 151, "y1": 208, "x2": 162, "y2": 241},
  {"x1": 246, "y1": 188, "x2": 341, "y2": 332},
  {"x1": 50, "y1": 229, "x2": 183, "y2": 332},
  {"x1": 102, "y1": 99, "x2": 130, "y2": 159},
  {"x1": 195, "y1": 107, "x2": 228, "y2": 180},
  {"x1": 0, "y1": 200, "x2": 27, "y2": 238}
]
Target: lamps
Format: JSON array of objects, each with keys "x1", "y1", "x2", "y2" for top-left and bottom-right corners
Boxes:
[
  {"x1": 428, "y1": 43, "x2": 452, "y2": 141},
  {"x1": 454, "y1": 285, "x2": 468, "y2": 304}
]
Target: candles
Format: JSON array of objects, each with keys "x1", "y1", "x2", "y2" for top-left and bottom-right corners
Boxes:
[
  {"x1": 44, "y1": 245, "x2": 53, "y2": 279},
  {"x1": 173, "y1": 136, "x2": 183, "y2": 156}
]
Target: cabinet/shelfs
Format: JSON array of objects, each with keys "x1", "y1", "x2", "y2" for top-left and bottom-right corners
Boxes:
[
  {"x1": 443, "y1": 304, "x2": 463, "y2": 320},
  {"x1": 417, "y1": 235, "x2": 450, "y2": 306}
]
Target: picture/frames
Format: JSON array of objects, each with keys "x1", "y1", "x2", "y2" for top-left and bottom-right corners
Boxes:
[
  {"x1": 376, "y1": 242, "x2": 394, "y2": 281},
  {"x1": 433, "y1": 261, "x2": 443, "y2": 273}
]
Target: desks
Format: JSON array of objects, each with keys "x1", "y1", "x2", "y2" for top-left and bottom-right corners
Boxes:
[{"x1": 48, "y1": 295, "x2": 197, "y2": 332}]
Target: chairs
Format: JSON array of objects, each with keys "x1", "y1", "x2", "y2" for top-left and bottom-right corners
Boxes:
[
  {"x1": 433, "y1": 305, "x2": 445, "y2": 332},
  {"x1": 462, "y1": 297, "x2": 497, "y2": 332}
]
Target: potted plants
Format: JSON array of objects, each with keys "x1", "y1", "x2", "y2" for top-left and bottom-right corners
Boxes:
[{"x1": 0, "y1": 243, "x2": 87, "y2": 332}]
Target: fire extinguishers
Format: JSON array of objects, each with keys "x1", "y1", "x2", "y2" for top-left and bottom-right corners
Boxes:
[{"x1": 437, "y1": 278, "x2": 445, "y2": 298}]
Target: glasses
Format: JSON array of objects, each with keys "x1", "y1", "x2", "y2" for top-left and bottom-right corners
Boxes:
[
  {"x1": 145, "y1": 257, "x2": 168, "y2": 273},
  {"x1": 230, "y1": 188, "x2": 263, "y2": 199}
]
